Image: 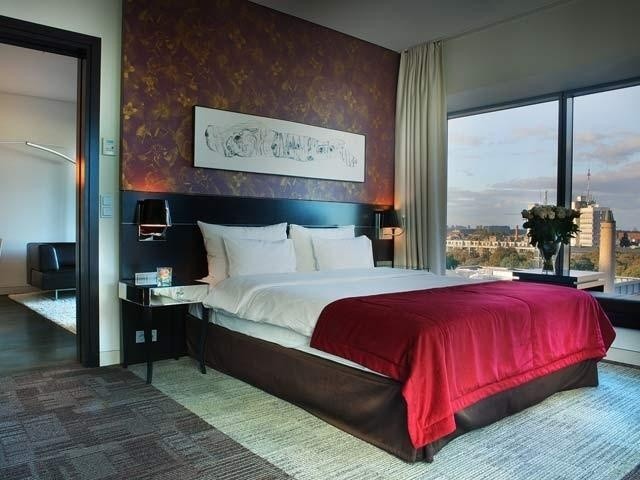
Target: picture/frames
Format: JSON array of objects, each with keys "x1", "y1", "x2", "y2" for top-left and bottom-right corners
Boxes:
[{"x1": 192, "y1": 105, "x2": 366, "y2": 183}]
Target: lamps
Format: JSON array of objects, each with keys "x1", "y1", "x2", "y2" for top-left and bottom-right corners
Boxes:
[
  {"x1": 134, "y1": 199, "x2": 173, "y2": 242},
  {"x1": 380, "y1": 210, "x2": 405, "y2": 235}
]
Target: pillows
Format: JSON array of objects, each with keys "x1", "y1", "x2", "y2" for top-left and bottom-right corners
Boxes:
[{"x1": 197, "y1": 221, "x2": 375, "y2": 281}]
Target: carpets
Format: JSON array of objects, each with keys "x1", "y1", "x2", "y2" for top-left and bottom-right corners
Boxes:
[
  {"x1": 124, "y1": 355, "x2": 640, "y2": 480},
  {"x1": 8, "y1": 291, "x2": 76, "y2": 334}
]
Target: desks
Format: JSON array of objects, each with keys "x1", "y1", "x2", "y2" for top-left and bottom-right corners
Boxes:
[
  {"x1": 513, "y1": 267, "x2": 605, "y2": 290},
  {"x1": 118, "y1": 279, "x2": 210, "y2": 385}
]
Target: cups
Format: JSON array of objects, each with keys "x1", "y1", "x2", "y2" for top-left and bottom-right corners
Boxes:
[{"x1": 156, "y1": 266, "x2": 173, "y2": 288}]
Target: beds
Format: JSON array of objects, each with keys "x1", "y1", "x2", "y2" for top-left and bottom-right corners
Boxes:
[{"x1": 186, "y1": 195, "x2": 599, "y2": 464}]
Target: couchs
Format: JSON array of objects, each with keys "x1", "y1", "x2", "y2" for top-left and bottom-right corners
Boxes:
[{"x1": 27, "y1": 243, "x2": 76, "y2": 300}]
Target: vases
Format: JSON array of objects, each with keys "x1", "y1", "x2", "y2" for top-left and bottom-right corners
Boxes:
[{"x1": 540, "y1": 240, "x2": 561, "y2": 272}]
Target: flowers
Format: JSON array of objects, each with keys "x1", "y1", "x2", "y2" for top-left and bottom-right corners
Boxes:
[{"x1": 521, "y1": 204, "x2": 584, "y2": 261}]
